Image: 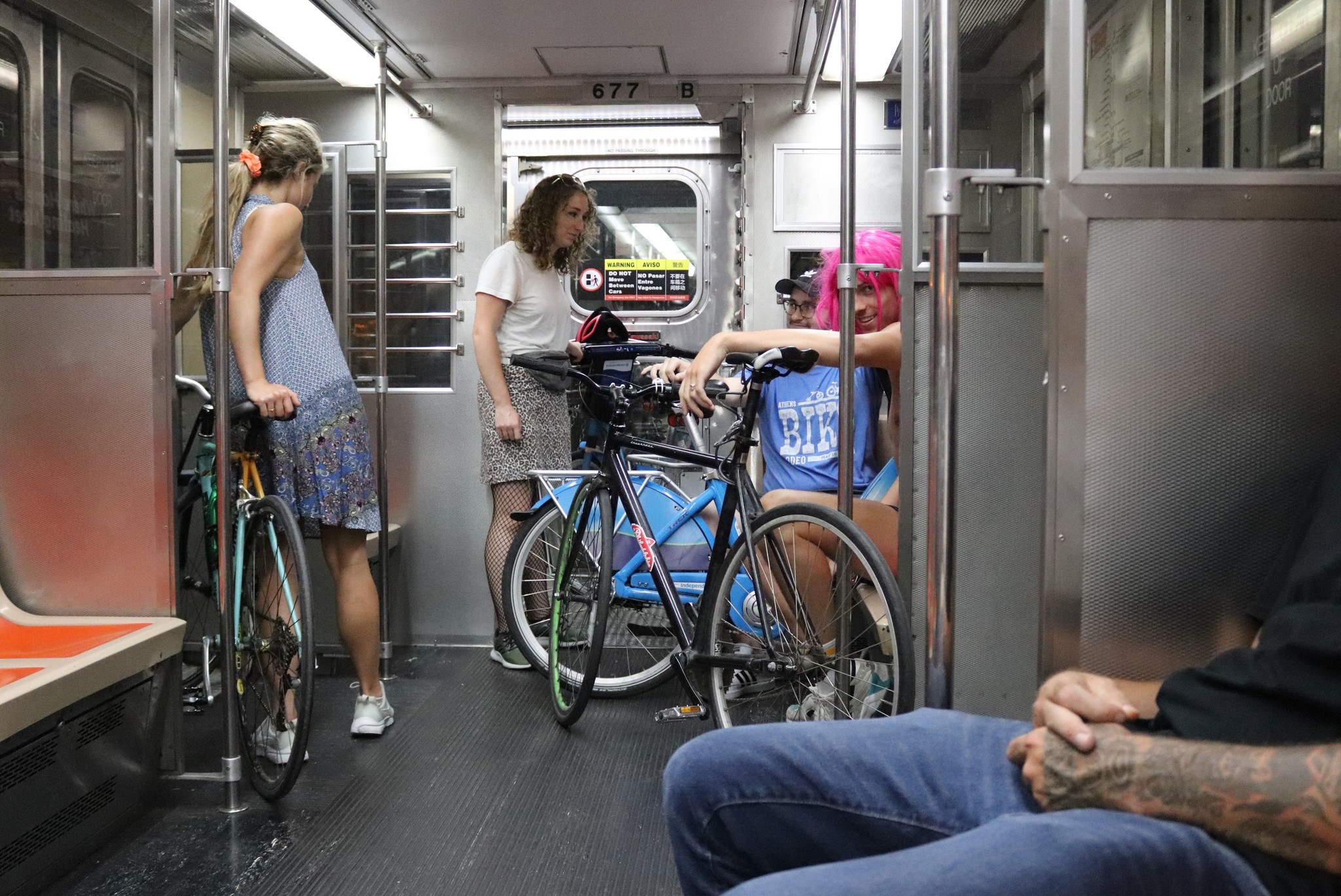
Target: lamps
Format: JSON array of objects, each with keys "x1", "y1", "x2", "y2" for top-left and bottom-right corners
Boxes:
[
  {"x1": 229, "y1": 0, "x2": 403, "y2": 89},
  {"x1": 819, "y1": 0, "x2": 903, "y2": 84}
]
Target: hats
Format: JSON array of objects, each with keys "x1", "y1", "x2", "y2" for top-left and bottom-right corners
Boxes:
[{"x1": 775, "y1": 268, "x2": 822, "y2": 298}]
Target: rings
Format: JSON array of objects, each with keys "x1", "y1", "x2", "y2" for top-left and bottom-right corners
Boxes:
[
  {"x1": 690, "y1": 383, "x2": 696, "y2": 391},
  {"x1": 268, "y1": 409, "x2": 275, "y2": 411}
]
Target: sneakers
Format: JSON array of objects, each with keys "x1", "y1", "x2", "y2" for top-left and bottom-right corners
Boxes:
[
  {"x1": 529, "y1": 620, "x2": 587, "y2": 648},
  {"x1": 786, "y1": 682, "x2": 817, "y2": 722},
  {"x1": 490, "y1": 628, "x2": 531, "y2": 669},
  {"x1": 252, "y1": 715, "x2": 309, "y2": 765},
  {"x1": 350, "y1": 678, "x2": 395, "y2": 734},
  {"x1": 808, "y1": 658, "x2": 890, "y2": 721},
  {"x1": 724, "y1": 642, "x2": 776, "y2": 698}
]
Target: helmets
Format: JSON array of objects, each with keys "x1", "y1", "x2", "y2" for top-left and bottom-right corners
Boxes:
[{"x1": 576, "y1": 306, "x2": 629, "y2": 344}]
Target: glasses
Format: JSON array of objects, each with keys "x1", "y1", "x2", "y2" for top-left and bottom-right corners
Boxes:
[
  {"x1": 552, "y1": 173, "x2": 586, "y2": 191},
  {"x1": 783, "y1": 300, "x2": 817, "y2": 318}
]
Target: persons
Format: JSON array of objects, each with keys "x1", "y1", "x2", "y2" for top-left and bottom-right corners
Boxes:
[
  {"x1": 172, "y1": 117, "x2": 395, "y2": 764},
  {"x1": 662, "y1": 444, "x2": 1341, "y2": 896},
  {"x1": 471, "y1": 173, "x2": 601, "y2": 669},
  {"x1": 640, "y1": 227, "x2": 903, "y2": 722}
]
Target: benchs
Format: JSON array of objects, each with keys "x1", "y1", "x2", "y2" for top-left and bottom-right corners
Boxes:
[{"x1": 0, "y1": 576, "x2": 188, "y2": 745}]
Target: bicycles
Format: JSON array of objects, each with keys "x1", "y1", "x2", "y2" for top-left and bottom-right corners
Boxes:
[
  {"x1": 510, "y1": 343, "x2": 915, "y2": 731},
  {"x1": 172, "y1": 375, "x2": 318, "y2": 803},
  {"x1": 498, "y1": 374, "x2": 895, "y2": 704}
]
